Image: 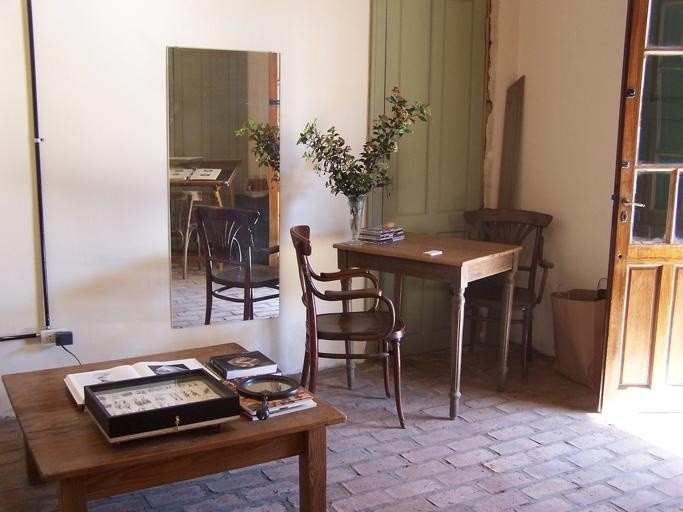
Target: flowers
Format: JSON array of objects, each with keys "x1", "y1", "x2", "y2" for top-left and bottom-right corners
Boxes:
[
  {"x1": 297, "y1": 86, "x2": 431, "y2": 226},
  {"x1": 234, "y1": 114, "x2": 280, "y2": 186}
]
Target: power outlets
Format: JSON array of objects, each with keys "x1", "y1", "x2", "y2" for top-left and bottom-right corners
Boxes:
[{"x1": 40, "y1": 329, "x2": 73, "y2": 345}]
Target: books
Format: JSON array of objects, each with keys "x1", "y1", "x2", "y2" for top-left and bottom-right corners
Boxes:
[
  {"x1": 224, "y1": 380, "x2": 317, "y2": 421},
  {"x1": 63, "y1": 358, "x2": 222, "y2": 408},
  {"x1": 356, "y1": 223, "x2": 405, "y2": 246},
  {"x1": 170, "y1": 166, "x2": 222, "y2": 183},
  {"x1": 209, "y1": 350, "x2": 277, "y2": 379},
  {"x1": 203, "y1": 362, "x2": 282, "y2": 383}
]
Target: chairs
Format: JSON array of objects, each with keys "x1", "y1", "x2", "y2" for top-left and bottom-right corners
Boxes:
[
  {"x1": 291, "y1": 225, "x2": 408, "y2": 428},
  {"x1": 195, "y1": 205, "x2": 280, "y2": 325},
  {"x1": 451, "y1": 208, "x2": 554, "y2": 383},
  {"x1": 171, "y1": 191, "x2": 208, "y2": 279}
]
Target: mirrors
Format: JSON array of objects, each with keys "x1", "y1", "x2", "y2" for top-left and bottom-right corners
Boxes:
[{"x1": 166, "y1": 46, "x2": 280, "y2": 329}]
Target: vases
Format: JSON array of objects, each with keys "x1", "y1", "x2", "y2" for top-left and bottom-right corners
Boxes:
[{"x1": 347, "y1": 195, "x2": 365, "y2": 246}]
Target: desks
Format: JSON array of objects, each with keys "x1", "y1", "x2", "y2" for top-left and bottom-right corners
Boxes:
[
  {"x1": 169, "y1": 159, "x2": 242, "y2": 280},
  {"x1": 0, "y1": 343, "x2": 347, "y2": 512},
  {"x1": 333, "y1": 231, "x2": 524, "y2": 420}
]
